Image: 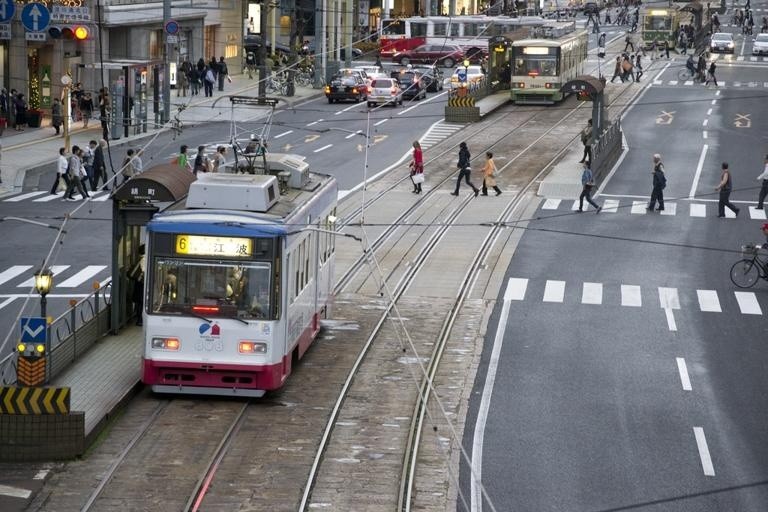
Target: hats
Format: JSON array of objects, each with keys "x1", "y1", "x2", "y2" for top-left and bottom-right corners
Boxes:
[{"x1": 759, "y1": 224, "x2": 768, "y2": 230}]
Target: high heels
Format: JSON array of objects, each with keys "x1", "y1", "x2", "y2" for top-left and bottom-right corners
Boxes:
[{"x1": 412, "y1": 188, "x2": 422, "y2": 194}]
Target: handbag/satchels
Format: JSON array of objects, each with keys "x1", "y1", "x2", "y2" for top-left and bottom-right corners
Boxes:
[{"x1": 412, "y1": 169, "x2": 424, "y2": 184}]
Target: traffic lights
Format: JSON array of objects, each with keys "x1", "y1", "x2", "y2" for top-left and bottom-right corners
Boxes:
[{"x1": 46, "y1": 24, "x2": 91, "y2": 41}]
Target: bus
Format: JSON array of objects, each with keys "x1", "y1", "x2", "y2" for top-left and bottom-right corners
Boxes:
[
  {"x1": 581, "y1": 0, "x2": 607, "y2": 16},
  {"x1": 641, "y1": 7, "x2": 680, "y2": 50},
  {"x1": 140, "y1": 149, "x2": 340, "y2": 404},
  {"x1": 510, "y1": 20, "x2": 590, "y2": 106},
  {"x1": 376, "y1": 14, "x2": 546, "y2": 64}
]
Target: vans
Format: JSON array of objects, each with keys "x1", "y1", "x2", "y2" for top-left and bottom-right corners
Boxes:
[{"x1": 367, "y1": 77, "x2": 403, "y2": 108}]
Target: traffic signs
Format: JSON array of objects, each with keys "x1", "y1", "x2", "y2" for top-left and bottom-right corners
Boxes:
[{"x1": 49, "y1": 5, "x2": 91, "y2": 23}]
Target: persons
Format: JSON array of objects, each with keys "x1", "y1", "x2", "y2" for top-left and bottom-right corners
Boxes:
[
  {"x1": 166, "y1": 270, "x2": 176, "y2": 295},
  {"x1": 478, "y1": 151, "x2": 502, "y2": 196},
  {"x1": 247, "y1": 49, "x2": 256, "y2": 79},
  {"x1": 576, "y1": 162, "x2": 601, "y2": 214},
  {"x1": 177, "y1": 56, "x2": 228, "y2": 97},
  {"x1": 645, "y1": 153, "x2": 666, "y2": 210},
  {"x1": 482, "y1": 55, "x2": 511, "y2": 89},
  {"x1": 177, "y1": 138, "x2": 259, "y2": 175},
  {"x1": 714, "y1": 163, "x2": 740, "y2": 217},
  {"x1": 585, "y1": 0, "x2": 767, "y2": 86},
  {"x1": 1, "y1": 83, "x2": 143, "y2": 201},
  {"x1": 451, "y1": 142, "x2": 480, "y2": 196},
  {"x1": 408, "y1": 141, "x2": 423, "y2": 193},
  {"x1": 755, "y1": 154, "x2": 768, "y2": 210},
  {"x1": 756, "y1": 223, "x2": 767, "y2": 279},
  {"x1": 130, "y1": 257, "x2": 145, "y2": 326},
  {"x1": 233, "y1": 267, "x2": 247, "y2": 307},
  {"x1": 264, "y1": 39, "x2": 310, "y2": 76},
  {"x1": 374, "y1": 55, "x2": 382, "y2": 68},
  {"x1": 580, "y1": 119, "x2": 593, "y2": 164}
]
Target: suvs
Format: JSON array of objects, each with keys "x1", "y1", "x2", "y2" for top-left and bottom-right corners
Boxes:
[
  {"x1": 390, "y1": 71, "x2": 427, "y2": 100},
  {"x1": 408, "y1": 65, "x2": 445, "y2": 91},
  {"x1": 393, "y1": 43, "x2": 463, "y2": 67}
]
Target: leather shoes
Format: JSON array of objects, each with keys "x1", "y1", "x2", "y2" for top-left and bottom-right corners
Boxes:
[
  {"x1": 451, "y1": 192, "x2": 458, "y2": 195},
  {"x1": 474, "y1": 188, "x2": 479, "y2": 196}
]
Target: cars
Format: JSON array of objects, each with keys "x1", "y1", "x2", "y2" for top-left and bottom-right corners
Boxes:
[
  {"x1": 710, "y1": 32, "x2": 735, "y2": 53},
  {"x1": 752, "y1": 32, "x2": 768, "y2": 55},
  {"x1": 326, "y1": 75, "x2": 367, "y2": 103},
  {"x1": 300, "y1": 39, "x2": 363, "y2": 62},
  {"x1": 452, "y1": 66, "x2": 486, "y2": 89},
  {"x1": 356, "y1": 65, "x2": 389, "y2": 79},
  {"x1": 335, "y1": 68, "x2": 373, "y2": 92},
  {"x1": 244, "y1": 34, "x2": 289, "y2": 65}
]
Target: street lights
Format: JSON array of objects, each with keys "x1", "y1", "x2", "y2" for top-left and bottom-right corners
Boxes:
[
  {"x1": 32, "y1": 257, "x2": 55, "y2": 317},
  {"x1": 61, "y1": 73, "x2": 73, "y2": 153},
  {"x1": 463, "y1": 58, "x2": 469, "y2": 85}
]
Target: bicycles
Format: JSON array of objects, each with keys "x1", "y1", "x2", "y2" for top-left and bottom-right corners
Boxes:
[
  {"x1": 262, "y1": 61, "x2": 313, "y2": 95},
  {"x1": 729, "y1": 243, "x2": 768, "y2": 290},
  {"x1": 679, "y1": 63, "x2": 705, "y2": 82}
]
